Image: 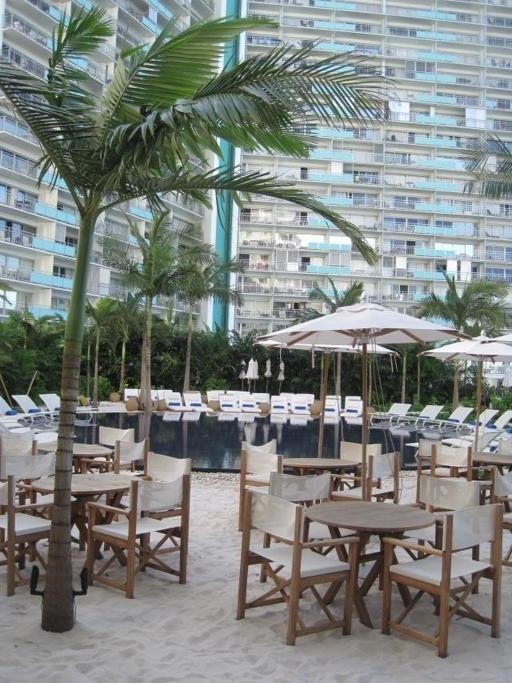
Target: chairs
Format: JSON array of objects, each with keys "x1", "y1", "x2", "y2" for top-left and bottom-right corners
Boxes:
[
  {"x1": 401, "y1": 472, "x2": 482, "y2": 596},
  {"x1": 122, "y1": 388, "x2": 364, "y2": 419},
  {"x1": 378, "y1": 501, "x2": 507, "y2": 657},
  {"x1": 368, "y1": 402, "x2": 512, "y2": 441},
  {"x1": 0, "y1": 393, "x2": 91, "y2": 424},
  {"x1": 259, "y1": 469, "x2": 336, "y2": 585},
  {"x1": 415, "y1": 439, "x2": 441, "y2": 502},
  {"x1": 235, "y1": 487, "x2": 356, "y2": 647},
  {"x1": 340, "y1": 440, "x2": 382, "y2": 490},
  {"x1": 490, "y1": 466, "x2": 511, "y2": 568},
  {"x1": 330, "y1": 451, "x2": 403, "y2": 507},
  {"x1": 430, "y1": 445, "x2": 474, "y2": 482},
  {"x1": 237, "y1": 436, "x2": 285, "y2": 532},
  {"x1": 0, "y1": 424, "x2": 191, "y2": 600}
]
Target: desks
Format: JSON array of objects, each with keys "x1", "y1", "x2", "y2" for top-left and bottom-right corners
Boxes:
[
  {"x1": 303, "y1": 499, "x2": 437, "y2": 630},
  {"x1": 281, "y1": 456, "x2": 357, "y2": 505},
  {"x1": 470, "y1": 450, "x2": 511, "y2": 467}
]
